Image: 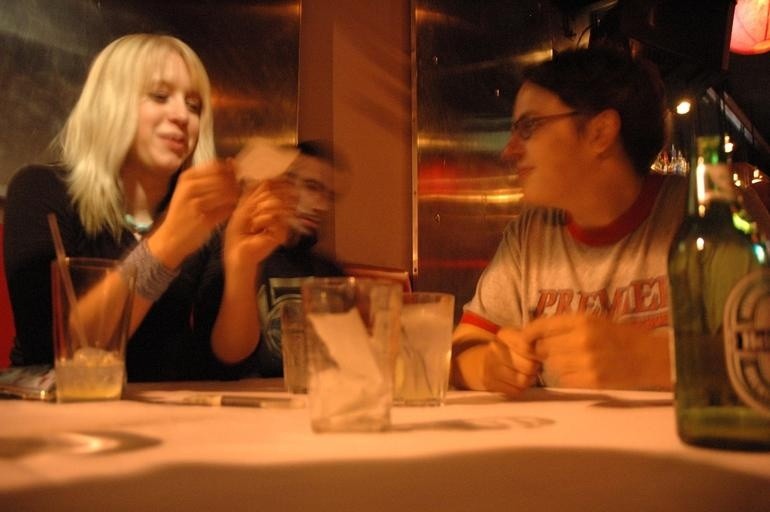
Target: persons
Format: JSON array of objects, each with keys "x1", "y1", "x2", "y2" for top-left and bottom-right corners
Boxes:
[
  {"x1": 248, "y1": 138, "x2": 346, "y2": 379},
  {"x1": 448, "y1": 43, "x2": 690, "y2": 395},
  {"x1": 6, "y1": 31, "x2": 301, "y2": 386}
]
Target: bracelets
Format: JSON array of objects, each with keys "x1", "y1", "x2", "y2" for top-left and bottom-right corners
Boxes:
[{"x1": 120, "y1": 239, "x2": 181, "y2": 306}]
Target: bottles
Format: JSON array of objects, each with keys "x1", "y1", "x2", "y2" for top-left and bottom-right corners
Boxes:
[{"x1": 668, "y1": 103, "x2": 770, "y2": 453}]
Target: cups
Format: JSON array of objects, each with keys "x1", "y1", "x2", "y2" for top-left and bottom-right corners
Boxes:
[
  {"x1": 49, "y1": 257, "x2": 133, "y2": 400},
  {"x1": 277, "y1": 277, "x2": 453, "y2": 433}
]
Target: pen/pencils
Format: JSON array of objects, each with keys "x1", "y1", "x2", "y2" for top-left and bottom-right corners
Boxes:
[{"x1": 185, "y1": 395, "x2": 262, "y2": 407}]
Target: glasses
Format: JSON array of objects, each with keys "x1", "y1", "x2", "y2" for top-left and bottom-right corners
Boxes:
[{"x1": 507, "y1": 108, "x2": 599, "y2": 142}]
